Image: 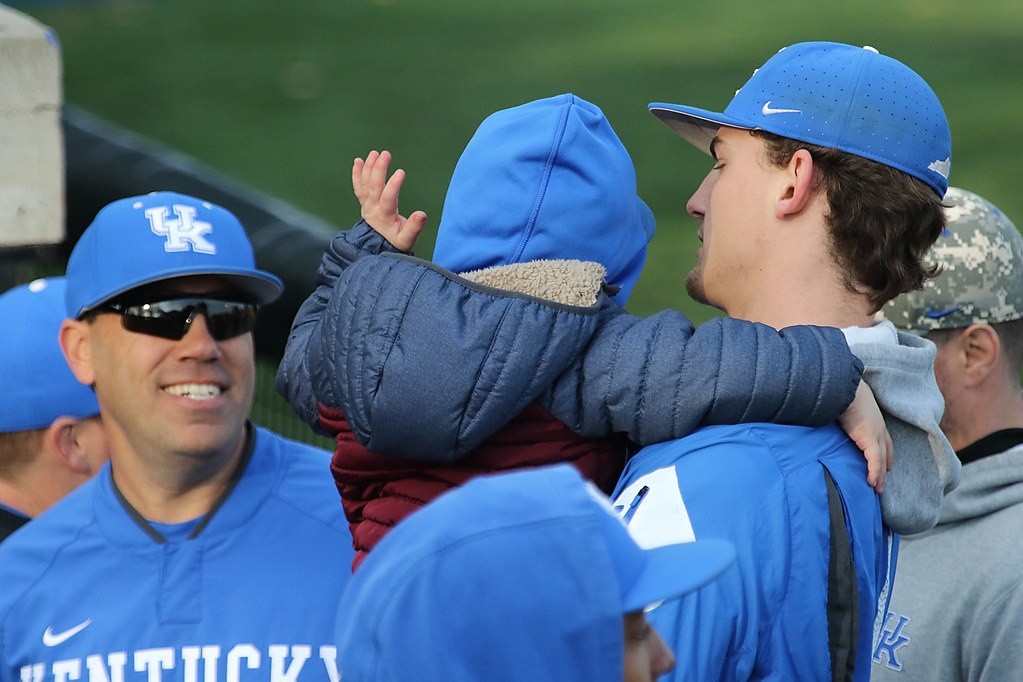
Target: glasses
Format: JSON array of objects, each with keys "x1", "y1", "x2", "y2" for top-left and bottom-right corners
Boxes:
[{"x1": 94, "y1": 289, "x2": 262, "y2": 340}]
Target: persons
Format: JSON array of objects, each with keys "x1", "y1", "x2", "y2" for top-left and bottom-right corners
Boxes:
[
  {"x1": 274, "y1": 92, "x2": 891, "y2": 563},
  {"x1": 0, "y1": 42, "x2": 1023, "y2": 682}
]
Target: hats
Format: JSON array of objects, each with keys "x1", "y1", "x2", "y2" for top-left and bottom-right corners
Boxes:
[
  {"x1": 881, "y1": 186, "x2": 1023, "y2": 336},
  {"x1": 0, "y1": 276, "x2": 100, "y2": 432},
  {"x1": 634, "y1": 195, "x2": 656, "y2": 247},
  {"x1": 64, "y1": 191, "x2": 283, "y2": 320},
  {"x1": 584, "y1": 481, "x2": 736, "y2": 615},
  {"x1": 647, "y1": 39, "x2": 952, "y2": 201}
]
